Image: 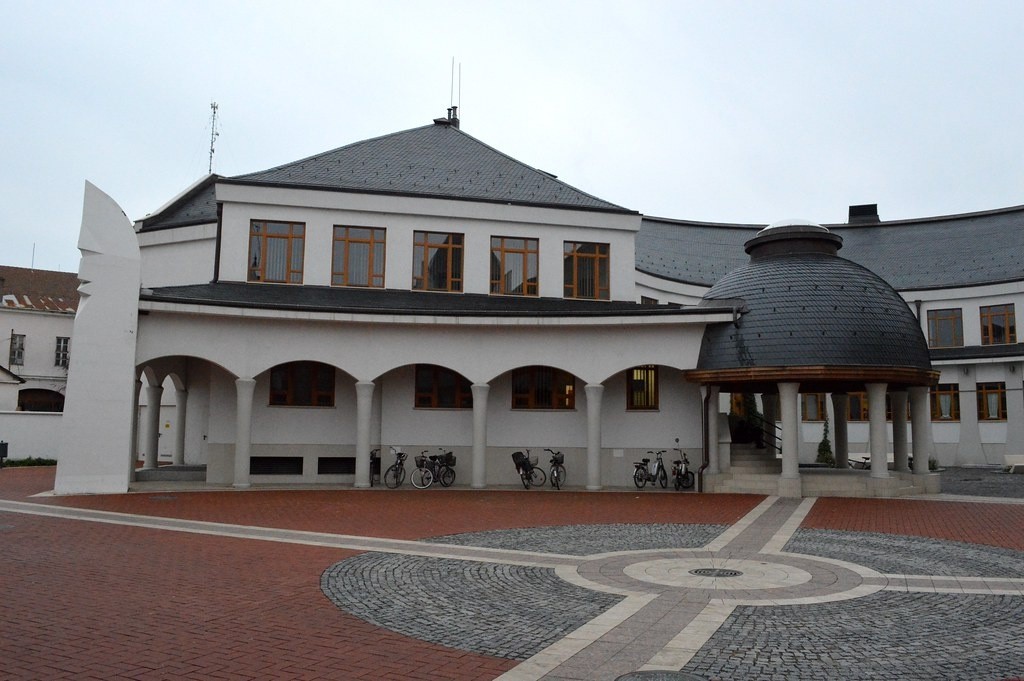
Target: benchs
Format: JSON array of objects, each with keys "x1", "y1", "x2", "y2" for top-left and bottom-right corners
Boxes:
[{"x1": 1003, "y1": 454, "x2": 1024, "y2": 473}]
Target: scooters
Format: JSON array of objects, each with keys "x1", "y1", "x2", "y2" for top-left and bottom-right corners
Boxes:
[
  {"x1": 632, "y1": 450, "x2": 668, "y2": 489},
  {"x1": 671, "y1": 437, "x2": 695, "y2": 491}
]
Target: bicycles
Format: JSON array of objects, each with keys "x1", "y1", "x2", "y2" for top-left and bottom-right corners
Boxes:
[
  {"x1": 370, "y1": 448, "x2": 381, "y2": 487},
  {"x1": 848, "y1": 457, "x2": 871, "y2": 471},
  {"x1": 511, "y1": 449, "x2": 547, "y2": 489},
  {"x1": 438, "y1": 447, "x2": 456, "y2": 487},
  {"x1": 544, "y1": 448, "x2": 567, "y2": 491},
  {"x1": 383, "y1": 446, "x2": 408, "y2": 489},
  {"x1": 410, "y1": 450, "x2": 441, "y2": 489}
]
